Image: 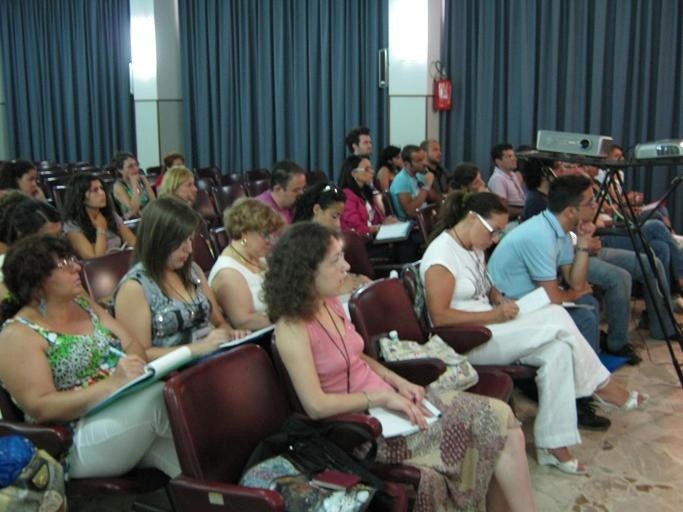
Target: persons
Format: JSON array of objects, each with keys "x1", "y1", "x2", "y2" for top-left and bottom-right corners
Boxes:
[{"x1": 3, "y1": 127, "x2": 682, "y2": 510}]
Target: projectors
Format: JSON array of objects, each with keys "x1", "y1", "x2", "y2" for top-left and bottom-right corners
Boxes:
[
  {"x1": 537, "y1": 129, "x2": 613, "y2": 158},
  {"x1": 635, "y1": 137, "x2": 683, "y2": 158}
]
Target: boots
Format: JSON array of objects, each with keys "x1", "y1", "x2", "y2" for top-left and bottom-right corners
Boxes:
[{"x1": 575, "y1": 396, "x2": 612, "y2": 430}]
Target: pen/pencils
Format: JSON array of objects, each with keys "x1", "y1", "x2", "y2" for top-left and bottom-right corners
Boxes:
[
  {"x1": 204, "y1": 317, "x2": 216, "y2": 331},
  {"x1": 502, "y1": 292, "x2": 510, "y2": 302},
  {"x1": 107, "y1": 344, "x2": 127, "y2": 357},
  {"x1": 421, "y1": 397, "x2": 442, "y2": 417}
]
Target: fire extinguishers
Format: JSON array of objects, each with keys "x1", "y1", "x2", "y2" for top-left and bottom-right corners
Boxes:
[{"x1": 433, "y1": 60, "x2": 452, "y2": 111}]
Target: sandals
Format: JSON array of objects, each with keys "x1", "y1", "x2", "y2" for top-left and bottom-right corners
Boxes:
[
  {"x1": 589, "y1": 375, "x2": 650, "y2": 413},
  {"x1": 535, "y1": 446, "x2": 590, "y2": 476}
]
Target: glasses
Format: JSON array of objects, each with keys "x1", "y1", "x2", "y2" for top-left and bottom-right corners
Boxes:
[
  {"x1": 352, "y1": 168, "x2": 375, "y2": 175},
  {"x1": 467, "y1": 208, "x2": 507, "y2": 242},
  {"x1": 320, "y1": 251, "x2": 345, "y2": 265},
  {"x1": 569, "y1": 195, "x2": 595, "y2": 208},
  {"x1": 48, "y1": 254, "x2": 78, "y2": 270}
]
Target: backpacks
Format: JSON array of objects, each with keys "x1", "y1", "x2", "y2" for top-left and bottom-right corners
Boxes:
[{"x1": 245, "y1": 405, "x2": 398, "y2": 512}]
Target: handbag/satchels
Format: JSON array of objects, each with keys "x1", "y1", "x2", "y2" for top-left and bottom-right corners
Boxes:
[
  {"x1": 380, "y1": 333, "x2": 480, "y2": 392},
  {"x1": 237, "y1": 454, "x2": 377, "y2": 511}
]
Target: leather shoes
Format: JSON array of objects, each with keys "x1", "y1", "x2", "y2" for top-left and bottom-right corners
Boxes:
[
  {"x1": 638, "y1": 297, "x2": 683, "y2": 342},
  {"x1": 605, "y1": 342, "x2": 643, "y2": 366}
]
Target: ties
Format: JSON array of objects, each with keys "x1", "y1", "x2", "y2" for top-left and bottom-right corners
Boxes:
[{"x1": 509, "y1": 177, "x2": 525, "y2": 201}]
